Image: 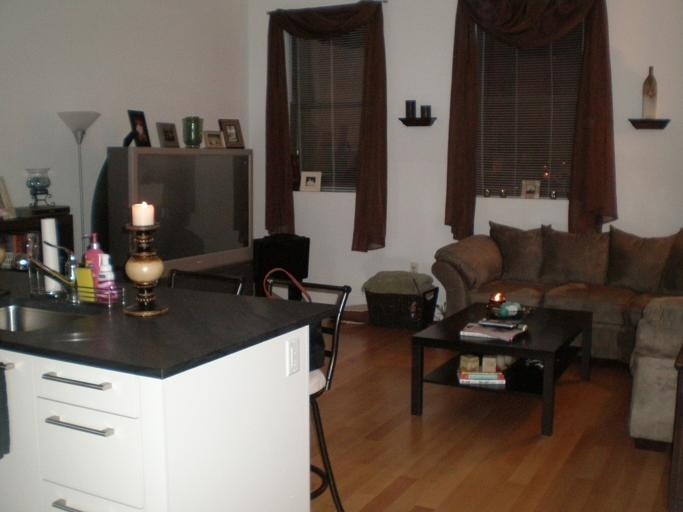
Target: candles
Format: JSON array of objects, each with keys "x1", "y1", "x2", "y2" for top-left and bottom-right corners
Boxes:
[{"x1": 132, "y1": 201, "x2": 155, "y2": 226}]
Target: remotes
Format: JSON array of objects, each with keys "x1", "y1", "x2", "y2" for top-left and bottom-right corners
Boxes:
[{"x1": 479, "y1": 317, "x2": 517, "y2": 329}]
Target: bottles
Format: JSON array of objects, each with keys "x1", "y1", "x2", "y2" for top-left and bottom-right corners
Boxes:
[
  {"x1": 497, "y1": 301, "x2": 520, "y2": 318},
  {"x1": 641, "y1": 64, "x2": 657, "y2": 120},
  {"x1": 64, "y1": 251, "x2": 80, "y2": 304}
]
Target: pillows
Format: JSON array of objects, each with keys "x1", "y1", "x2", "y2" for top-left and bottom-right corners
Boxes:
[{"x1": 488, "y1": 222, "x2": 683, "y2": 295}]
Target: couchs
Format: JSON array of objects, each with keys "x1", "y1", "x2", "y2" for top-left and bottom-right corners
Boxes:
[{"x1": 432, "y1": 235, "x2": 683, "y2": 452}]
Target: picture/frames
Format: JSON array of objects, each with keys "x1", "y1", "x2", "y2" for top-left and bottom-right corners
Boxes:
[
  {"x1": 156, "y1": 122, "x2": 179, "y2": 148},
  {"x1": 124, "y1": 110, "x2": 151, "y2": 147},
  {"x1": 300, "y1": 171, "x2": 321, "y2": 192},
  {"x1": 204, "y1": 131, "x2": 223, "y2": 148},
  {"x1": 218, "y1": 119, "x2": 244, "y2": 148},
  {"x1": 521, "y1": 180, "x2": 540, "y2": 199}
]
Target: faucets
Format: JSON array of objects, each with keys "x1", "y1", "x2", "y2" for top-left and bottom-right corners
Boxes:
[{"x1": 27, "y1": 241, "x2": 81, "y2": 307}]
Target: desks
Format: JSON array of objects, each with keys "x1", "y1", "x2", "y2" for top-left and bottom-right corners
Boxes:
[{"x1": 0, "y1": 208, "x2": 73, "y2": 271}]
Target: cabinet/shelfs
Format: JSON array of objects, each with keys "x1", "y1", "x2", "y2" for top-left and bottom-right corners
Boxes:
[{"x1": 0, "y1": 323, "x2": 310, "y2": 512}]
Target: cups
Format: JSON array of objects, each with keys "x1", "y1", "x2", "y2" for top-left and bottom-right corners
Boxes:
[
  {"x1": 499, "y1": 188, "x2": 506, "y2": 197},
  {"x1": 419, "y1": 105, "x2": 431, "y2": 117},
  {"x1": 483, "y1": 189, "x2": 490, "y2": 196},
  {"x1": 405, "y1": 100, "x2": 416, "y2": 119},
  {"x1": 548, "y1": 189, "x2": 558, "y2": 198}
]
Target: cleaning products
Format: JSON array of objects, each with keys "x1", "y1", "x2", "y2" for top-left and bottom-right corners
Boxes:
[{"x1": 81, "y1": 232, "x2": 104, "y2": 304}]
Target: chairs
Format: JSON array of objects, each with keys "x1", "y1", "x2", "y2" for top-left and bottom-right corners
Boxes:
[
  {"x1": 266, "y1": 278, "x2": 351, "y2": 512},
  {"x1": 170, "y1": 269, "x2": 243, "y2": 296}
]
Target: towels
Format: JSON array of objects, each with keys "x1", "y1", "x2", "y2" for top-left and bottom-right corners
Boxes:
[{"x1": 0, "y1": 363, "x2": 10, "y2": 458}]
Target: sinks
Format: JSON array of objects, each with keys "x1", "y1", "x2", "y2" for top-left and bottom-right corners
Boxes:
[{"x1": 0, "y1": 295, "x2": 105, "y2": 336}]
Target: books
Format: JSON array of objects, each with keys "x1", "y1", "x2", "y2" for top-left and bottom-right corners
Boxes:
[
  {"x1": 6, "y1": 233, "x2": 25, "y2": 253},
  {"x1": 457, "y1": 369, "x2": 506, "y2": 385},
  {"x1": 460, "y1": 322, "x2": 527, "y2": 342}
]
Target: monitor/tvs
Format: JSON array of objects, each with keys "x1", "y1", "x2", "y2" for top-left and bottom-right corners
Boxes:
[{"x1": 91, "y1": 148, "x2": 253, "y2": 285}]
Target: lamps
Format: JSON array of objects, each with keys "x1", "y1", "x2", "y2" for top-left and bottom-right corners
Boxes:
[{"x1": 57, "y1": 111, "x2": 102, "y2": 268}]
